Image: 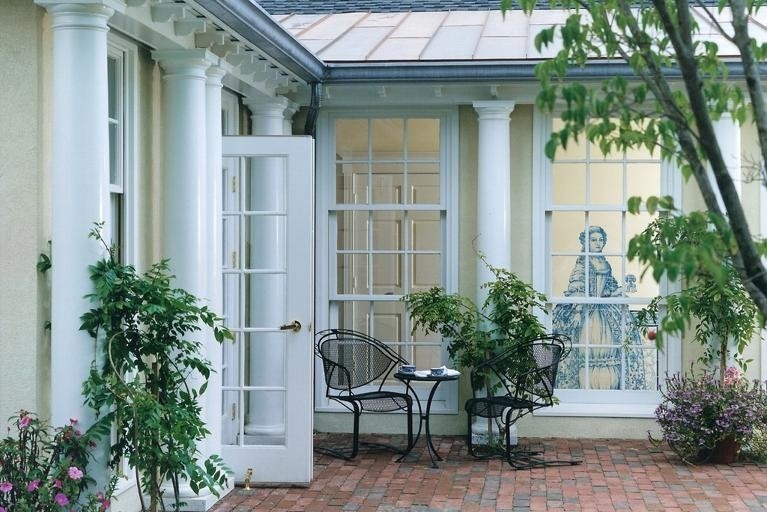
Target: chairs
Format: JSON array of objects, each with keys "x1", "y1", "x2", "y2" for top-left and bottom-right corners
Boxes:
[
  {"x1": 466, "y1": 335, "x2": 582, "y2": 469},
  {"x1": 314, "y1": 329, "x2": 413, "y2": 458}
]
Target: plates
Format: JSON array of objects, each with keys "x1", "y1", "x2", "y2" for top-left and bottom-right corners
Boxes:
[{"x1": 426, "y1": 373, "x2": 449, "y2": 378}]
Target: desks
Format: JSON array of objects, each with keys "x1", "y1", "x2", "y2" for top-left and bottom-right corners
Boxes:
[{"x1": 394, "y1": 371, "x2": 461, "y2": 468}]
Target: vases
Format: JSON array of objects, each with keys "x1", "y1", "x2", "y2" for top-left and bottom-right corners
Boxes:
[{"x1": 712, "y1": 436, "x2": 740, "y2": 463}]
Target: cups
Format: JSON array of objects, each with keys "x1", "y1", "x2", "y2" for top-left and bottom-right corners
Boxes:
[
  {"x1": 430, "y1": 367, "x2": 447, "y2": 375},
  {"x1": 398, "y1": 364, "x2": 416, "y2": 375}
]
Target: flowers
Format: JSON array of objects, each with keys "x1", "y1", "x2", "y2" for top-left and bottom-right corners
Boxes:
[{"x1": 647, "y1": 363, "x2": 767, "y2": 467}]
